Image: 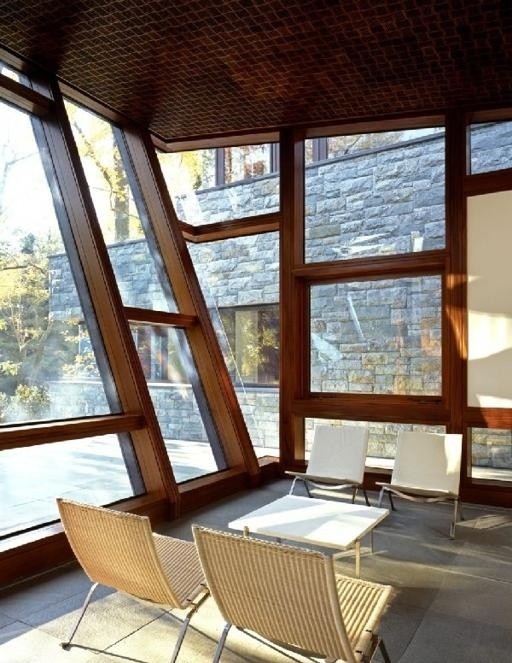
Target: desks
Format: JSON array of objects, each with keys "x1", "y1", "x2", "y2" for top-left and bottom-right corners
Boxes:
[{"x1": 225, "y1": 493, "x2": 390, "y2": 579}]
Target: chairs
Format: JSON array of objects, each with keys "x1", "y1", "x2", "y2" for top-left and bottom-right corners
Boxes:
[
  {"x1": 191, "y1": 524, "x2": 395, "y2": 663},
  {"x1": 56, "y1": 500, "x2": 209, "y2": 663},
  {"x1": 284, "y1": 421, "x2": 371, "y2": 504},
  {"x1": 374, "y1": 431, "x2": 465, "y2": 538}
]
